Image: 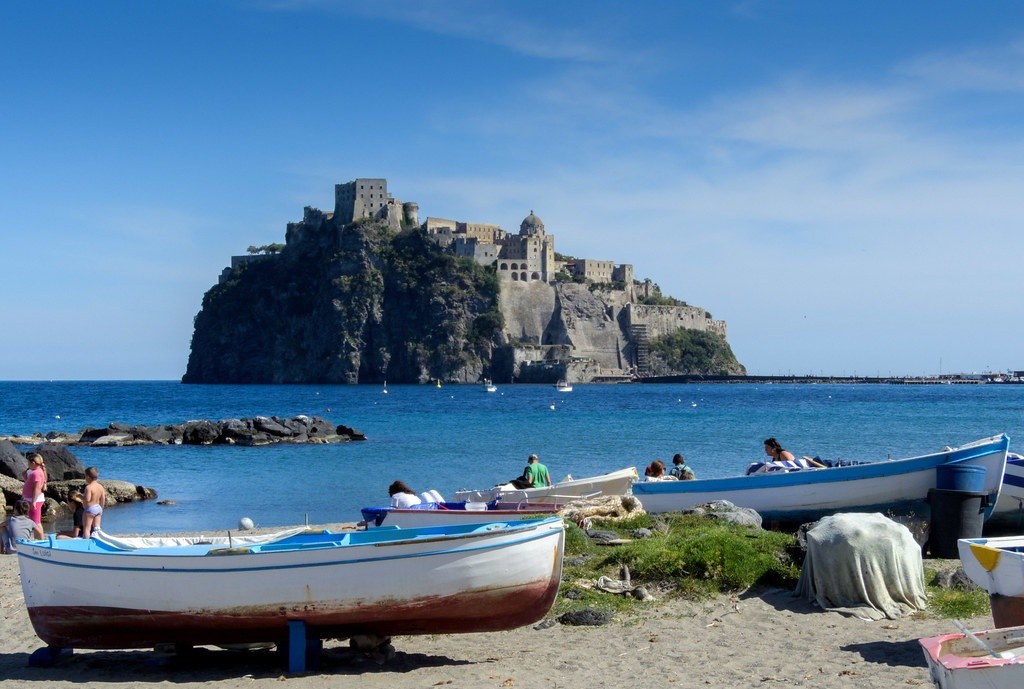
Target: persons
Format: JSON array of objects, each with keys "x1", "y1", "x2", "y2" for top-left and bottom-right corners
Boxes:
[
  {"x1": 668, "y1": 453, "x2": 694, "y2": 481},
  {"x1": 644, "y1": 458, "x2": 665, "y2": 478},
  {"x1": 21, "y1": 453, "x2": 47, "y2": 541},
  {"x1": 764, "y1": 438, "x2": 795, "y2": 462},
  {"x1": 55, "y1": 489, "x2": 95, "y2": 540},
  {"x1": 518, "y1": 454, "x2": 551, "y2": 489},
  {"x1": 0, "y1": 500, "x2": 43, "y2": 555},
  {"x1": 74, "y1": 467, "x2": 105, "y2": 539},
  {"x1": 388, "y1": 480, "x2": 421, "y2": 509}
]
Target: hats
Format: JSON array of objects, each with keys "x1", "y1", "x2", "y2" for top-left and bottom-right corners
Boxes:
[{"x1": 527, "y1": 454, "x2": 538, "y2": 463}]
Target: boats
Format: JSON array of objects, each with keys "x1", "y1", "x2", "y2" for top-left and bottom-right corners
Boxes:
[
  {"x1": 634, "y1": 432, "x2": 1011, "y2": 526},
  {"x1": 957, "y1": 537, "x2": 1024, "y2": 628},
  {"x1": 919, "y1": 625, "x2": 1024, "y2": 689},
  {"x1": 361, "y1": 502, "x2": 567, "y2": 527},
  {"x1": 15, "y1": 517, "x2": 566, "y2": 647},
  {"x1": 455, "y1": 466, "x2": 639, "y2": 502}
]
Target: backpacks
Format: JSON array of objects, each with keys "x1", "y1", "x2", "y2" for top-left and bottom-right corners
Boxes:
[{"x1": 676, "y1": 467, "x2": 692, "y2": 480}]
